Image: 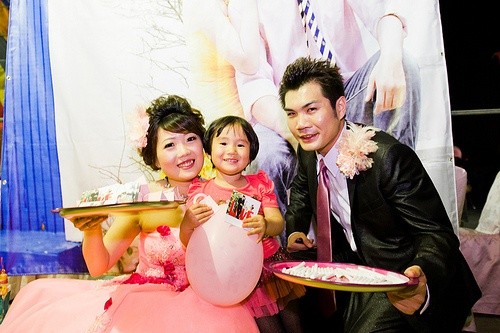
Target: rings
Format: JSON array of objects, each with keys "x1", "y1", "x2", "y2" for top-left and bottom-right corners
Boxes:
[{"x1": 86, "y1": 225, "x2": 90, "y2": 228}]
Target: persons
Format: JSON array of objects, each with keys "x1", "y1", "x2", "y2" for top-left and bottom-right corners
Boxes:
[
  {"x1": 128, "y1": 0, "x2": 422, "y2": 213},
  {"x1": 180, "y1": 115, "x2": 307, "y2": 333},
  {"x1": 244, "y1": 204, "x2": 254, "y2": 218},
  {"x1": 236, "y1": 195, "x2": 245, "y2": 219},
  {"x1": 278, "y1": 55, "x2": 482, "y2": 333},
  {"x1": 69, "y1": 94, "x2": 261, "y2": 333}
]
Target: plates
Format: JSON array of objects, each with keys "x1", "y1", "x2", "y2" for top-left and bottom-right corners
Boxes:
[
  {"x1": 262, "y1": 259, "x2": 419, "y2": 292},
  {"x1": 51, "y1": 199, "x2": 184, "y2": 218}
]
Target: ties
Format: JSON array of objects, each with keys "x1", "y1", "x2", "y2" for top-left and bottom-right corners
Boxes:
[
  {"x1": 297, "y1": 0, "x2": 338, "y2": 68},
  {"x1": 316, "y1": 158, "x2": 332, "y2": 262}
]
